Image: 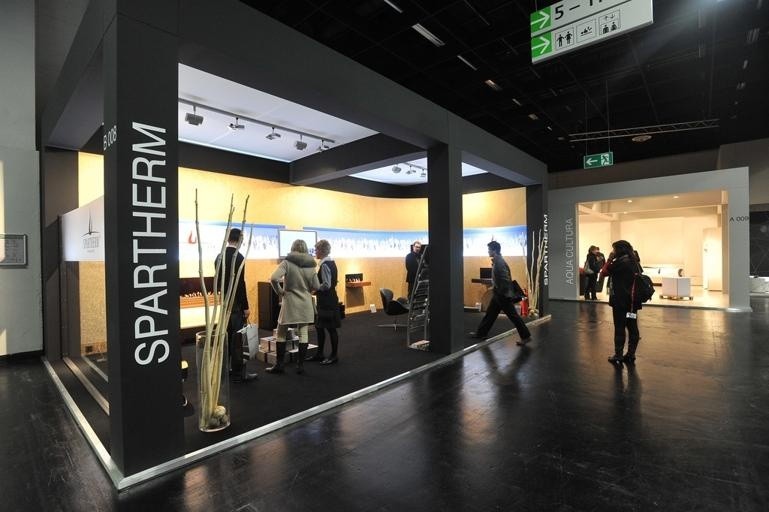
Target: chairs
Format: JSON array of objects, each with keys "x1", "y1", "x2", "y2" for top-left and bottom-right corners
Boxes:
[{"x1": 376, "y1": 287, "x2": 409, "y2": 331}]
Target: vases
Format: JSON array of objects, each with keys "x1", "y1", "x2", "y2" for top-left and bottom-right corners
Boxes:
[
  {"x1": 527, "y1": 282, "x2": 541, "y2": 320},
  {"x1": 196, "y1": 333, "x2": 230, "y2": 432}
]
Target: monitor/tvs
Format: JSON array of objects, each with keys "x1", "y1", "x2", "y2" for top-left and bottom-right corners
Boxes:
[{"x1": 278, "y1": 229, "x2": 317, "y2": 261}]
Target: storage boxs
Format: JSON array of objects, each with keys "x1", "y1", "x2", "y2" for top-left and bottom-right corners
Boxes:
[{"x1": 256, "y1": 327, "x2": 318, "y2": 366}]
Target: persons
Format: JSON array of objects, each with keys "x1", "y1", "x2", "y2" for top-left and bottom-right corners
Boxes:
[
  {"x1": 264, "y1": 239, "x2": 321, "y2": 375},
  {"x1": 304, "y1": 239, "x2": 342, "y2": 366},
  {"x1": 214, "y1": 227, "x2": 259, "y2": 385},
  {"x1": 405, "y1": 241, "x2": 423, "y2": 305},
  {"x1": 582, "y1": 239, "x2": 644, "y2": 368},
  {"x1": 469, "y1": 240, "x2": 534, "y2": 346}
]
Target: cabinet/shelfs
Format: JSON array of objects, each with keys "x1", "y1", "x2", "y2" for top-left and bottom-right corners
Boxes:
[
  {"x1": 659, "y1": 277, "x2": 693, "y2": 301},
  {"x1": 258, "y1": 280, "x2": 317, "y2": 330}
]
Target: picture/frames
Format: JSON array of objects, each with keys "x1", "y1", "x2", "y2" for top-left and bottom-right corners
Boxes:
[{"x1": 0, "y1": 234, "x2": 28, "y2": 268}]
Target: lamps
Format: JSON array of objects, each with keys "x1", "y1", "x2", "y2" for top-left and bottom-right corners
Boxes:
[
  {"x1": 175, "y1": 99, "x2": 335, "y2": 153},
  {"x1": 392, "y1": 164, "x2": 426, "y2": 177}
]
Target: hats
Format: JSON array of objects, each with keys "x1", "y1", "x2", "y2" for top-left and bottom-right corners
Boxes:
[{"x1": 588, "y1": 246, "x2": 598, "y2": 254}]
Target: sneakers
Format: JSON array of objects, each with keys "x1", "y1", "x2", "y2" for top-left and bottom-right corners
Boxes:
[
  {"x1": 516, "y1": 336, "x2": 532, "y2": 346},
  {"x1": 468, "y1": 330, "x2": 480, "y2": 339}
]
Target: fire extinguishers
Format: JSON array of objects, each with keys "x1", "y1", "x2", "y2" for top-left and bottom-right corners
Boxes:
[{"x1": 520, "y1": 288, "x2": 529, "y2": 319}]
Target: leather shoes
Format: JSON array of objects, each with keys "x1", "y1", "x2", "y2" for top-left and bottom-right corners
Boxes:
[{"x1": 230, "y1": 369, "x2": 259, "y2": 383}]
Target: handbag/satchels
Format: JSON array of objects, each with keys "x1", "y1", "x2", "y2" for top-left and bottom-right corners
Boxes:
[
  {"x1": 511, "y1": 280, "x2": 526, "y2": 304},
  {"x1": 634, "y1": 260, "x2": 656, "y2": 304}
]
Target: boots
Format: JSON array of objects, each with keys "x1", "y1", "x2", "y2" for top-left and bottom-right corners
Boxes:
[
  {"x1": 608, "y1": 336, "x2": 626, "y2": 369},
  {"x1": 265, "y1": 341, "x2": 287, "y2": 375},
  {"x1": 304, "y1": 336, "x2": 325, "y2": 362},
  {"x1": 623, "y1": 337, "x2": 640, "y2": 367},
  {"x1": 294, "y1": 342, "x2": 309, "y2": 374},
  {"x1": 319, "y1": 336, "x2": 338, "y2": 368}
]
